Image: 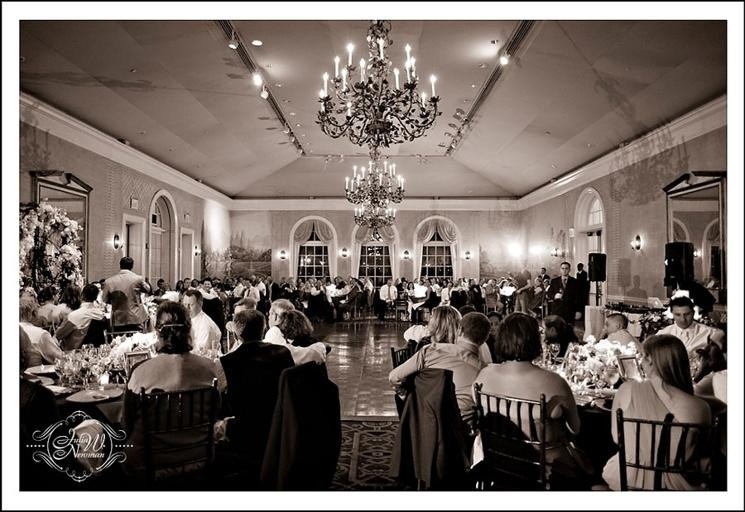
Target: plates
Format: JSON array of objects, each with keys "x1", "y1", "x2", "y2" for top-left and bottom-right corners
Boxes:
[
  {"x1": 65, "y1": 389, "x2": 110, "y2": 403},
  {"x1": 21, "y1": 376, "x2": 54, "y2": 386},
  {"x1": 24, "y1": 364, "x2": 56, "y2": 375},
  {"x1": 595, "y1": 397, "x2": 612, "y2": 411}
]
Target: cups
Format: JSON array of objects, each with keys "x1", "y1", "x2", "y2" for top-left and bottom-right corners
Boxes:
[
  {"x1": 54, "y1": 357, "x2": 68, "y2": 387},
  {"x1": 559, "y1": 288, "x2": 564, "y2": 301},
  {"x1": 99, "y1": 344, "x2": 112, "y2": 377},
  {"x1": 81, "y1": 344, "x2": 95, "y2": 368},
  {"x1": 603, "y1": 366, "x2": 620, "y2": 397},
  {"x1": 89, "y1": 348, "x2": 99, "y2": 379},
  {"x1": 76, "y1": 360, "x2": 90, "y2": 398},
  {"x1": 549, "y1": 343, "x2": 560, "y2": 370}
]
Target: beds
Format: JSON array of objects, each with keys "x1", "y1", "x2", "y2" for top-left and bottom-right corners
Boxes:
[{"x1": 20, "y1": 356, "x2": 218, "y2": 434}]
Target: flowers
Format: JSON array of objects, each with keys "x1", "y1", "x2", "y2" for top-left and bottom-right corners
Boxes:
[{"x1": 19, "y1": 197, "x2": 84, "y2": 293}]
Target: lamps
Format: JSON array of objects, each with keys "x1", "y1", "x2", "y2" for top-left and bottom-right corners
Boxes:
[
  {"x1": 192, "y1": 245, "x2": 204, "y2": 258},
  {"x1": 110, "y1": 234, "x2": 124, "y2": 253},
  {"x1": 400, "y1": 250, "x2": 412, "y2": 261},
  {"x1": 288, "y1": 133, "x2": 304, "y2": 154},
  {"x1": 457, "y1": 250, "x2": 476, "y2": 260},
  {"x1": 251, "y1": 39, "x2": 263, "y2": 48},
  {"x1": 276, "y1": 248, "x2": 290, "y2": 265},
  {"x1": 629, "y1": 235, "x2": 643, "y2": 253},
  {"x1": 550, "y1": 245, "x2": 566, "y2": 260},
  {"x1": 436, "y1": 107, "x2": 469, "y2": 155},
  {"x1": 259, "y1": 86, "x2": 268, "y2": 100},
  {"x1": 227, "y1": 27, "x2": 240, "y2": 51},
  {"x1": 283, "y1": 122, "x2": 289, "y2": 134},
  {"x1": 316, "y1": 20, "x2": 442, "y2": 246},
  {"x1": 497, "y1": 48, "x2": 514, "y2": 68},
  {"x1": 337, "y1": 246, "x2": 351, "y2": 259},
  {"x1": 250, "y1": 65, "x2": 263, "y2": 88}
]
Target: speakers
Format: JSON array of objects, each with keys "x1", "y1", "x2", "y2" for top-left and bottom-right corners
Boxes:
[
  {"x1": 664, "y1": 242, "x2": 693, "y2": 290},
  {"x1": 588, "y1": 253, "x2": 606, "y2": 283}
]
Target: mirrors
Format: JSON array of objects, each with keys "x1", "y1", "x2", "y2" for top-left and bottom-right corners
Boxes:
[
  {"x1": 664, "y1": 176, "x2": 725, "y2": 294},
  {"x1": 38, "y1": 182, "x2": 86, "y2": 282}
]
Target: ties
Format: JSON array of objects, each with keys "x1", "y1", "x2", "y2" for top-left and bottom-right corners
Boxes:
[
  {"x1": 387, "y1": 286, "x2": 391, "y2": 300},
  {"x1": 561, "y1": 278, "x2": 567, "y2": 290}
]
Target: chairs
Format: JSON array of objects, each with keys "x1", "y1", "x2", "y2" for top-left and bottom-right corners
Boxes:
[
  {"x1": 615, "y1": 408, "x2": 727, "y2": 492},
  {"x1": 393, "y1": 291, "x2": 409, "y2": 324},
  {"x1": 239, "y1": 359, "x2": 340, "y2": 490},
  {"x1": 390, "y1": 339, "x2": 428, "y2": 372},
  {"x1": 124, "y1": 377, "x2": 222, "y2": 490},
  {"x1": 398, "y1": 366, "x2": 468, "y2": 492},
  {"x1": 473, "y1": 382, "x2": 596, "y2": 493}
]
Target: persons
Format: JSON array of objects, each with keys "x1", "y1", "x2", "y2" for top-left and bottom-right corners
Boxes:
[
  {"x1": 483, "y1": 311, "x2": 503, "y2": 363},
  {"x1": 429, "y1": 305, "x2": 461, "y2": 343},
  {"x1": 102, "y1": 257, "x2": 154, "y2": 332},
  {"x1": 263, "y1": 299, "x2": 295, "y2": 345},
  {"x1": 576, "y1": 262, "x2": 587, "y2": 280},
  {"x1": 655, "y1": 297, "x2": 723, "y2": 377},
  {"x1": 601, "y1": 333, "x2": 712, "y2": 491},
  {"x1": 692, "y1": 342, "x2": 727, "y2": 395},
  {"x1": 54, "y1": 281, "x2": 81, "y2": 323},
  {"x1": 455, "y1": 305, "x2": 493, "y2": 365},
  {"x1": 36, "y1": 285, "x2": 58, "y2": 323},
  {"x1": 56, "y1": 283, "x2": 102, "y2": 351},
  {"x1": 541, "y1": 314, "x2": 579, "y2": 359},
  {"x1": 19, "y1": 296, "x2": 64, "y2": 366},
  {"x1": 279, "y1": 310, "x2": 332, "y2": 364},
  {"x1": 154, "y1": 268, "x2": 556, "y2": 323},
  {"x1": 388, "y1": 313, "x2": 488, "y2": 464},
  {"x1": 182, "y1": 290, "x2": 222, "y2": 359},
  {"x1": 403, "y1": 308, "x2": 431, "y2": 342},
  {"x1": 593, "y1": 313, "x2": 643, "y2": 356},
  {"x1": 548, "y1": 263, "x2": 585, "y2": 328},
  {"x1": 662, "y1": 274, "x2": 716, "y2": 316},
  {"x1": 470, "y1": 312, "x2": 587, "y2": 485},
  {"x1": 226, "y1": 297, "x2": 258, "y2": 353},
  {"x1": 215, "y1": 311, "x2": 295, "y2": 447},
  {"x1": 123, "y1": 301, "x2": 227, "y2": 467}
]
